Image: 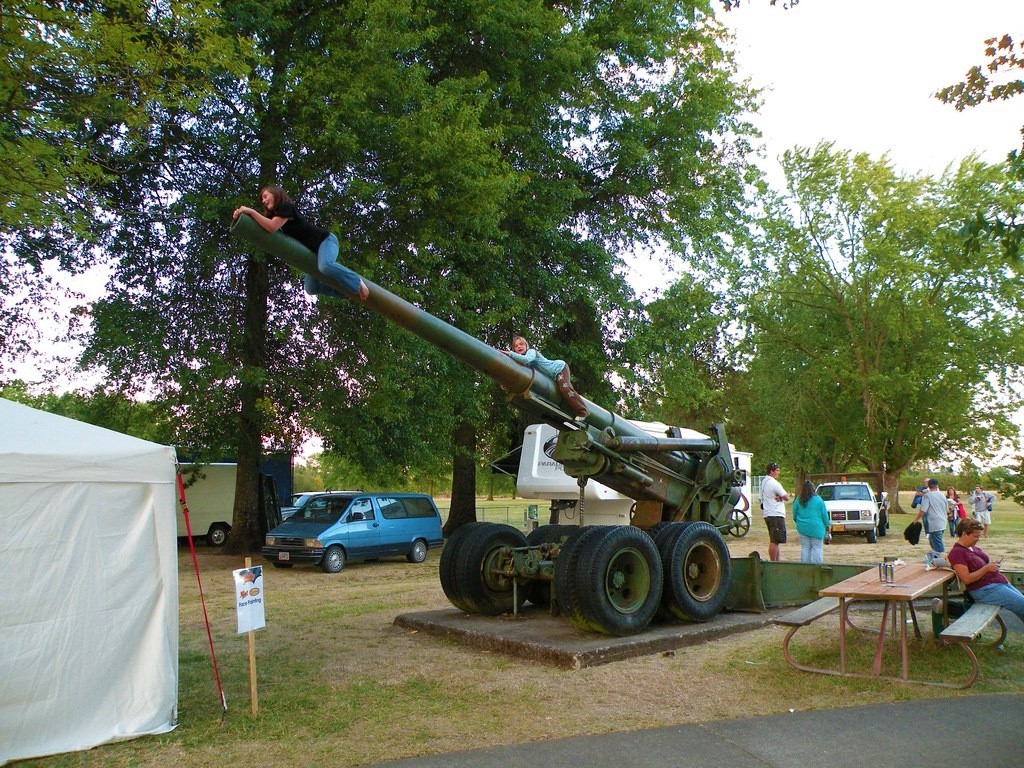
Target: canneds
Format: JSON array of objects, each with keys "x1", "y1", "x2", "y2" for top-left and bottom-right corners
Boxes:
[
  {"x1": 879, "y1": 563, "x2": 887, "y2": 582},
  {"x1": 885, "y1": 564, "x2": 894, "y2": 583},
  {"x1": 927, "y1": 551, "x2": 934, "y2": 567}
]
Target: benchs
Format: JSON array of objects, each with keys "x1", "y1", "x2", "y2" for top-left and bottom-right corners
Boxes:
[
  {"x1": 772, "y1": 596, "x2": 852, "y2": 677},
  {"x1": 938, "y1": 602, "x2": 1007, "y2": 690}
]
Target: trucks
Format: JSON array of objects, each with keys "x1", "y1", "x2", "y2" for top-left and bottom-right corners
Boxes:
[{"x1": 175, "y1": 447, "x2": 293, "y2": 506}]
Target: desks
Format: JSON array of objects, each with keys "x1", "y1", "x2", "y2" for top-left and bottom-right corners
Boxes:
[{"x1": 818, "y1": 561, "x2": 957, "y2": 683}]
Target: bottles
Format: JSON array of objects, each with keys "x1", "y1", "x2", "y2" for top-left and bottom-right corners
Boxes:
[
  {"x1": 532, "y1": 517, "x2": 538, "y2": 533},
  {"x1": 925, "y1": 552, "x2": 933, "y2": 568},
  {"x1": 825, "y1": 530, "x2": 830, "y2": 544}
]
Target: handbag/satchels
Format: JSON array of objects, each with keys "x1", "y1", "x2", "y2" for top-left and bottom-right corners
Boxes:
[
  {"x1": 761, "y1": 503, "x2": 763, "y2": 509},
  {"x1": 987, "y1": 498, "x2": 992, "y2": 511}
]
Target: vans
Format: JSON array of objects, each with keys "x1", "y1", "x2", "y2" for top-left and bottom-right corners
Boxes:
[
  {"x1": 280, "y1": 491, "x2": 364, "y2": 521},
  {"x1": 262, "y1": 493, "x2": 445, "y2": 573},
  {"x1": 176, "y1": 463, "x2": 238, "y2": 547}
]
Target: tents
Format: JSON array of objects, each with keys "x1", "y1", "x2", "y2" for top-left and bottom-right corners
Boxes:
[{"x1": 0, "y1": 397, "x2": 176, "y2": 768}]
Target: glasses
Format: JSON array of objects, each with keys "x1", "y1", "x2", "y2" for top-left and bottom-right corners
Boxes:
[
  {"x1": 772, "y1": 462, "x2": 777, "y2": 467},
  {"x1": 973, "y1": 524, "x2": 982, "y2": 528}
]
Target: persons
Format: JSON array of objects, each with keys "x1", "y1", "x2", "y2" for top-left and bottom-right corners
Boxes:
[
  {"x1": 233, "y1": 186, "x2": 369, "y2": 300},
  {"x1": 911, "y1": 478, "x2": 939, "y2": 539},
  {"x1": 500, "y1": 336, "x2": 591, "y2": 421},
  {"x1": 914, "y1": 479, "x2": 948, "y2": 553},
  {"x1": 759, "y1": 462, "x2": 789, "y2": 561},
  {"x1": 947, "y1": 516, "x2": 1024, "y2": 622},
  {"x1": 969, "y1": 486, "x2": 997, "y2": 538},
  {"x1": 946, "y1": 487, "x2": 966, "y2": 539},
  {"x1": 792, "y1": 481, "x2": 832, "y2": 564}
]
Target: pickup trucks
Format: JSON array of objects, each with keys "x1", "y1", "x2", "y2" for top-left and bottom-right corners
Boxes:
[{"x1": 815, "y1": 477, "x2": 886, "y2": 543}]
